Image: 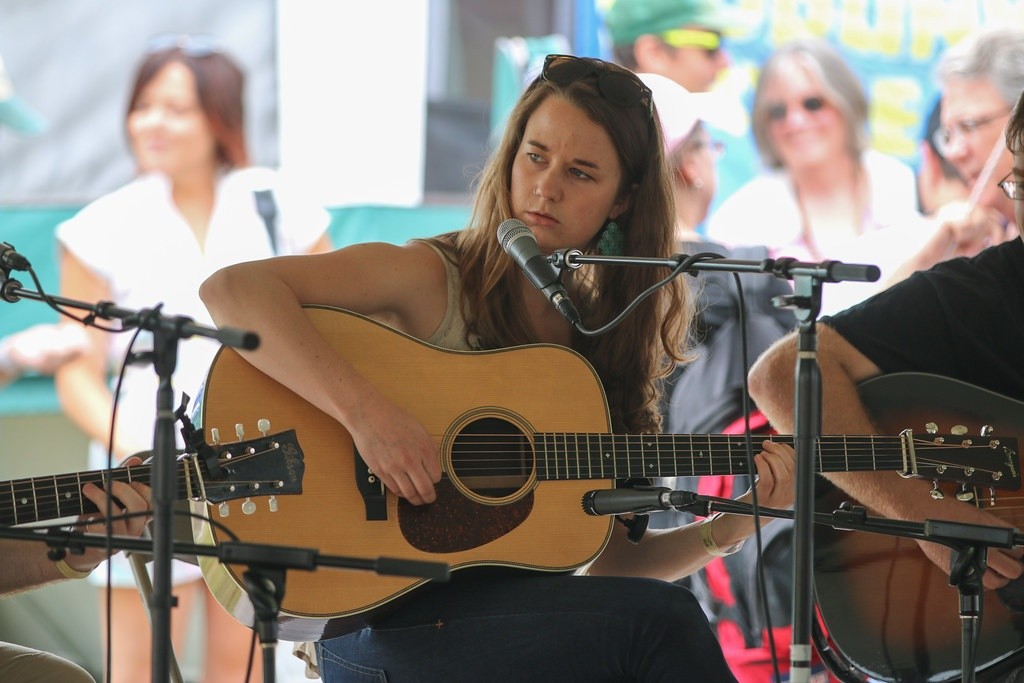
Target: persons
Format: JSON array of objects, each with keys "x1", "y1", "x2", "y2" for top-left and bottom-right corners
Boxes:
[
  {"x1": 598, "y1": 0, "x2": 1024, "y2": 683},
  {"x1": 198, "y1": 52, "x2": 798, "y2": 683},
  {"x1": 0, "y1": 457, "x2": 155, "y2": 683},
  {"x1": 0, "y1": 321, "x2": 88, "y2": 387},
  {"x1": 55, "y1": 38, "x2": 334, "y2": 683}
]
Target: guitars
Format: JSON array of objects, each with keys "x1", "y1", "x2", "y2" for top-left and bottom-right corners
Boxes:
[
  {"x1": 0, "y1": 417, "x2": 306, "y2": 530},
  {"x1": 810, "y1": 371, "x2": 1024, "y2": 683},
  {"x1": 189, "y1": 304, "x2": 1022, "y2": 643}
]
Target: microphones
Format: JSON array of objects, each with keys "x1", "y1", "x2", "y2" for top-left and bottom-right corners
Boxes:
[
  {"x1": 0, "y1": 242, "x2": 32, "y2": 271},
  {"x1": 497, "y1": 219, "x2": 581, "y2": 326},
  {"x1": 580, "y1": 489, "x2": 691, "y2": 517}
]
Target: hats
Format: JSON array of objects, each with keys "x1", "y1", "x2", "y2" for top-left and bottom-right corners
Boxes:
[
  {"x1": 611, "y1": 0, "x2": 727, "y2": 44},
  {"x1": 0, "y1": 55, "x2": 52, "y2": 135},
  {"x1": 632, "y1": 72, "x2": 721, "y2": 157}
]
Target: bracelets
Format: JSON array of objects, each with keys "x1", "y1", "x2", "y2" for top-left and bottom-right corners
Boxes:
[{"x1": 700, "y1": 512, "x2": 745, "y2": 557}]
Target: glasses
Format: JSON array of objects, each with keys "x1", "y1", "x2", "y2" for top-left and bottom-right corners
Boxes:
[
  {"x1": 146, "y1": 32, "x2": 222, "y2": 58},
  {"x1": 528, "y1": 54, "x2": 653, "y2": 144},
  {"x1": 678, "y1": 141, "x2": 725, "y2": 169},
  {"x1": 932, "y1": 106, "x2": 1018, "y2": 158},
  {"x1": 661, "y1": 28, "x2": 720, "y2": 55},
  {"x1": 997, "y1": 172, "x2": 1024, "y2": 200},
  {"x1": 765, "y1": 91, "x2": 825, "y2": 122}
]
